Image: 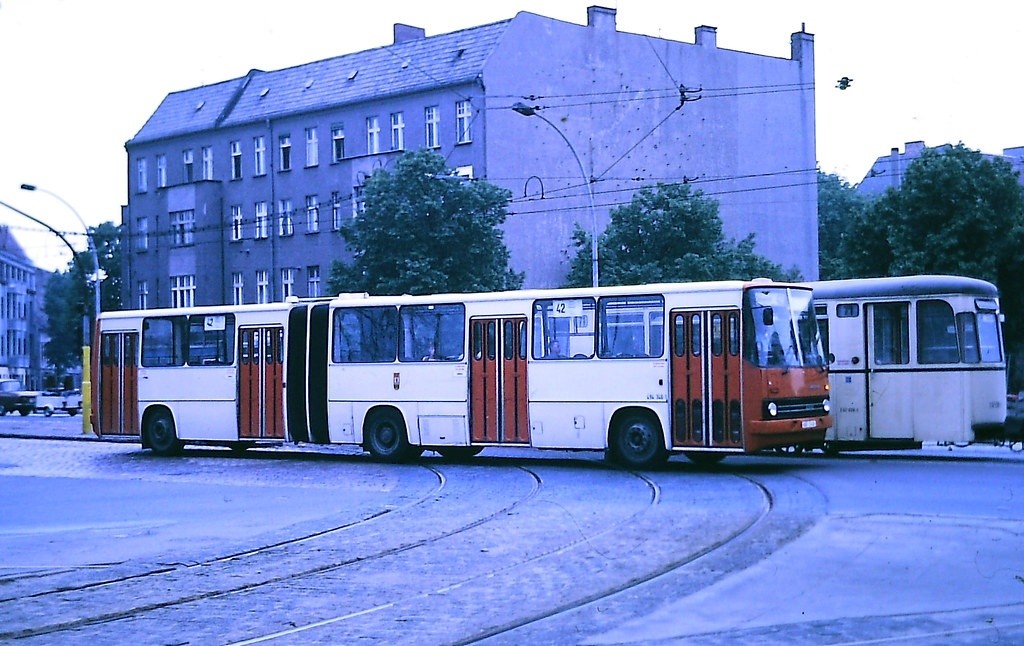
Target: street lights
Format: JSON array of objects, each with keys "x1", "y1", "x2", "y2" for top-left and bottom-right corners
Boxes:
[
  {"x1": 19, "y1": 184, "x2": 100, "y2": 315},
  {"x1": 510, "y1": 102, "x2": 601, "y2": 288}
]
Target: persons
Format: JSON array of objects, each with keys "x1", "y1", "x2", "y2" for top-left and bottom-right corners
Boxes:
[
  {"x1": 543, "y1": 340, "x2": 561, "y2": 360},
  {"x1": 423, "y1": 342, "x2": 441, "y2": 362}
]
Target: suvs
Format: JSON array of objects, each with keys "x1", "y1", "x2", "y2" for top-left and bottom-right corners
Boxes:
[{"x1": 0, "y1": 380, "x2": 36, "y2": 416}]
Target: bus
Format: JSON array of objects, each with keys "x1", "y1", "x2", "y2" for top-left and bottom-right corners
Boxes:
[{"x1": 512, "y1": 275, "x2": 1008, "y2": 458}]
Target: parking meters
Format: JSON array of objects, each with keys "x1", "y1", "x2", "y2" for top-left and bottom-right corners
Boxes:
[{"x1": 92, "y1": 275, "x2": 836, "y2": 473}]
